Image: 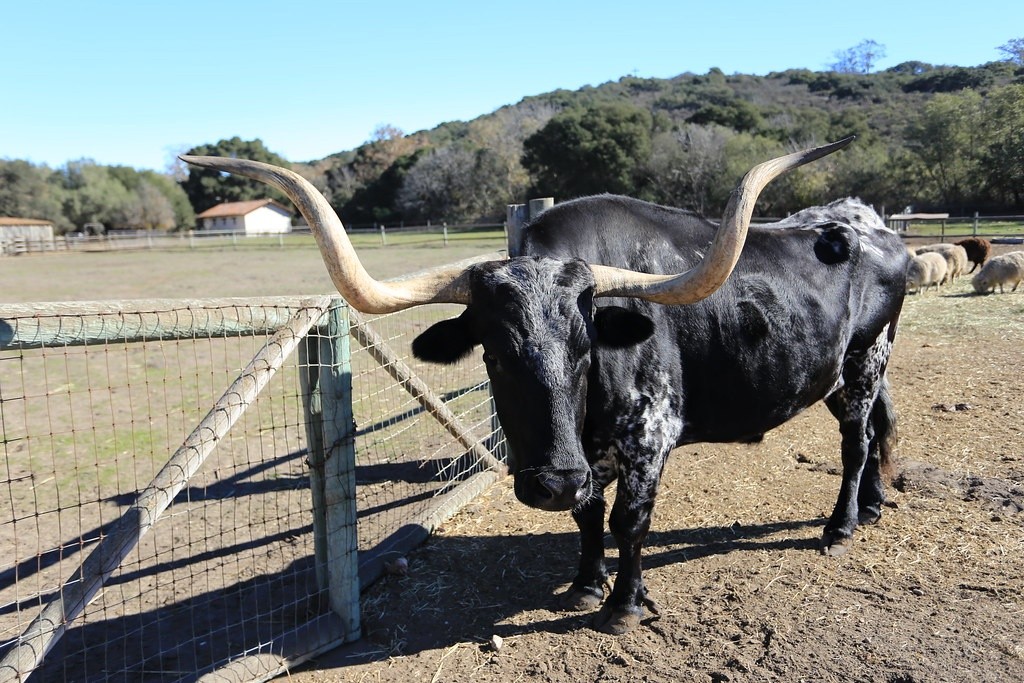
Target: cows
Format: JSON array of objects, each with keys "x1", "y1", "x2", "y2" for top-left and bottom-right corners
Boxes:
[{"x1": 175, "y1": 128, "x2": 918, "y2": 636}]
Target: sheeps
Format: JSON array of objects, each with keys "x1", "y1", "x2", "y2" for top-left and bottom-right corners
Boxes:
[
  {"x1": 907, "y1": 237, "x2": 990, "y2": 294},
  {"x1": 969, "y1": 251, "x2": 1023, "y2": 294}
]
